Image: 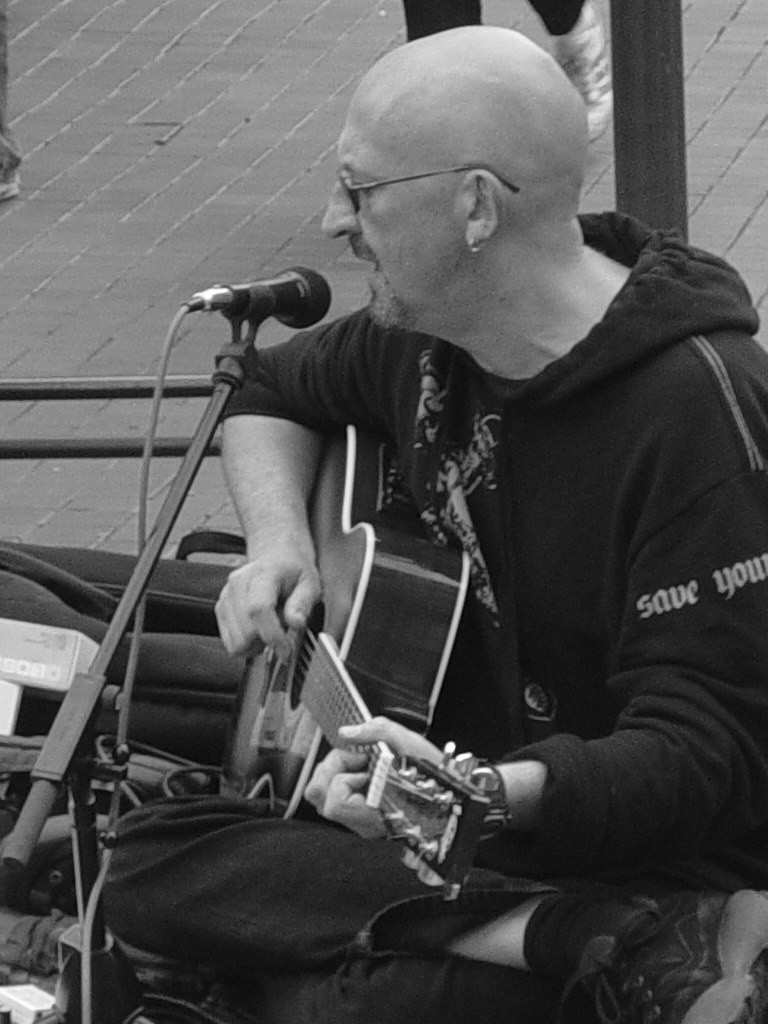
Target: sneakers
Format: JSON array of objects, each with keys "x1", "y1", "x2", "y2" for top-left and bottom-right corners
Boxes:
[{"x1": 556, "y1": 886, "x2": 768, "y2": 1024}]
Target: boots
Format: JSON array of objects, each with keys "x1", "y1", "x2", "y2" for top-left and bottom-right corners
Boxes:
[{"x1": 548, "y1": 1, "x2": 613, "y2": 143}]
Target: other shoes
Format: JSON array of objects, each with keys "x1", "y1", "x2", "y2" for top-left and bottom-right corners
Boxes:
[{"x1": 0, "y1": 169, "x2": 21, "y2": 201}]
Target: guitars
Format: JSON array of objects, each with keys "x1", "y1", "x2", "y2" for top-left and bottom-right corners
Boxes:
[{"x1": 218, "y1": 420, "x2": 510, "y2": 900}]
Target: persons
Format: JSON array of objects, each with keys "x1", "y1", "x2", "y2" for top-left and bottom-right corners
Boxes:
[
  {"x1": 402, "y1": 0, "x2": 614, "y2": 144},
  {"x1": 102, "y1": 26, "x2": 768, "y2": 1024},
  {"x1": 0, "y1": 0, "x2": 22, "y2": 201}
]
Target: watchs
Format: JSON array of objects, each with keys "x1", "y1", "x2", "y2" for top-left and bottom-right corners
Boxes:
[{"x1": 477, "y1": 763, "x2": 513, "y2": 839}]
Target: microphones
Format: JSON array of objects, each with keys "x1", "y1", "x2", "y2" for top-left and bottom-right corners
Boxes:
[{"x1": 180, "y1": 266, "x2": 332, "y2": 329}]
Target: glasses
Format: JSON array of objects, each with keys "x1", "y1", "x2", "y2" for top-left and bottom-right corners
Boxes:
[{"x1": 337, "y1": 163, "x2": 518, "y2": 214}]
